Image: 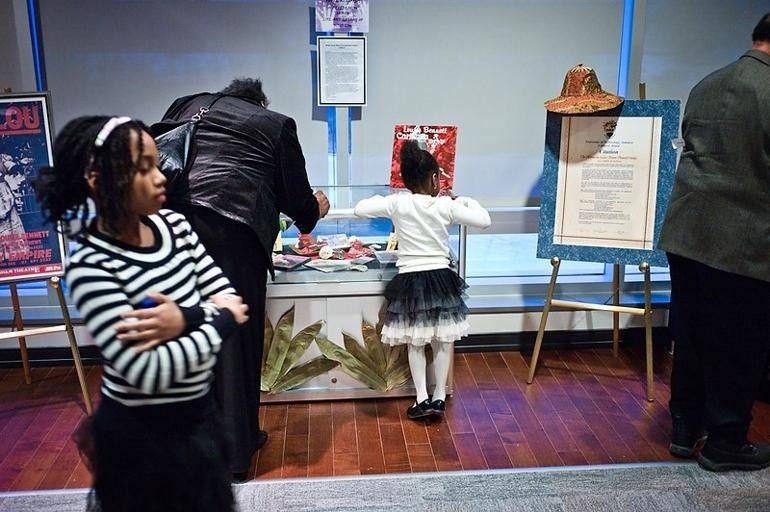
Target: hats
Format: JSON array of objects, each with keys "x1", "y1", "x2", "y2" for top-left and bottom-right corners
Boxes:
[{"x1": 544, "y1": 63, "x2": 624, "y2": 114}]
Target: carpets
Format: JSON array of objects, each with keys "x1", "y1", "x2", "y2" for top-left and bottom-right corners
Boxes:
[{"x1": 0, "y1": 462, "x2": 768, "y2": 512}]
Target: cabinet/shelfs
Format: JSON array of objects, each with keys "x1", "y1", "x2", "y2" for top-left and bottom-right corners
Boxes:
[{"x1": 259, "y1": 237, "x2": 456, "y2": 405}]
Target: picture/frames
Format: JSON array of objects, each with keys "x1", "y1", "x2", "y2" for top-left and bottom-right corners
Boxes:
[{"x1": 1, "y1": 90, "x2": 67, "y2": 283}]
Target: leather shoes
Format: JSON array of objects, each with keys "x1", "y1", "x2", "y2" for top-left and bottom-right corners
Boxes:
[
  {"x1": 406, "y1": 399, "x2": 432, "y2": 419},
  {"x1": 234, "y1": 430, "x2": 268, "y2": 480},
  {"x1": 433, "y1": 400, "x2": 446, "y2": 415}
]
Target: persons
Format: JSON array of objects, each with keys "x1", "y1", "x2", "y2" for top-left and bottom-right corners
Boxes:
[
  {"x1": 29, "y1": 115, "x2": 250, "y2": 512},
  {"x1": 353, "y1": 142, "x2": 492, "y2": 419},
  {"x1": 316, "y1": 0, "x2": 369, "y2": 32},
  {"x1": 656, "y1": 11, "x2": 770, "y2": 473},
  {"x1": 160, "y1": 78, "x2": 330, "y2": 456}
]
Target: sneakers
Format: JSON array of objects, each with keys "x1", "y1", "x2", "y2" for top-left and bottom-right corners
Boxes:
[
  {"x1": 698, "y1": 439, "x2": 770, "y2": 472},
  {"x1": 670, "y1": 419, "x2": 708, "y2": 456}
]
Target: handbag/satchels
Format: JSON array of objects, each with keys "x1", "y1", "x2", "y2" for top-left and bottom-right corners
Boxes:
[{"x1": 146, "y1": 119, "x2": 195, "y2": 186}]
